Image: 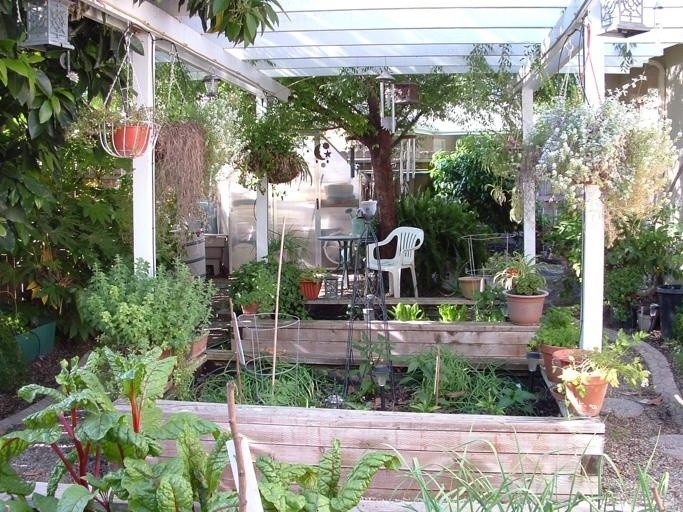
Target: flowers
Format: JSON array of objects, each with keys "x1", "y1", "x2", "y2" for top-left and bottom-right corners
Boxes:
[{"x1": 492, "y1": 249, "x2": 551, "y2": 296}]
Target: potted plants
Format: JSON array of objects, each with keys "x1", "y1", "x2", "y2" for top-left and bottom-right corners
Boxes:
[
  {"x1": 233, "y1": 91, "x2": 312, "y2": 198},
  {"x1": 548, "y1": 321, "x2": 660, "y2": 420},
  {"x1": 67, "y1": 246, "x2": 218, "y2": 398},
  {"x1": 295, "y1": 266, "x2": 323, "y2": 300},
  {"x1": 94, "y1": 100, "x2": 154, "y2": 157},
  {"x1": 521, "y1": 304, "x2": 584, "y2": 382},
  {"x1": 164, "y1": 269, "x2": 215, "y2": 362}
]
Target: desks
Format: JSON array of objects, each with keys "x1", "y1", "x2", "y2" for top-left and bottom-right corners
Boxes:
[{"x1": 317, "y1": 235, "x2": 372, "y2": 296}]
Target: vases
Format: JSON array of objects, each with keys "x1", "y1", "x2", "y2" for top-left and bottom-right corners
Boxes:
[
  {"x1": 551, "y1": 345, "x2": 598, "y2": 374},
  {"x1": 455, "y1": 275, "x2": 484, "y2": 298},
  {"x1": 501, "y1": 290, "x2": 548, "y2": 326}
]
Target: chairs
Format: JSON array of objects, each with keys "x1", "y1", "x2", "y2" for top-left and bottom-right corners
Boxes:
[{"x1": 365, "y1": 226, "x2": 424, "y2": 298}]
